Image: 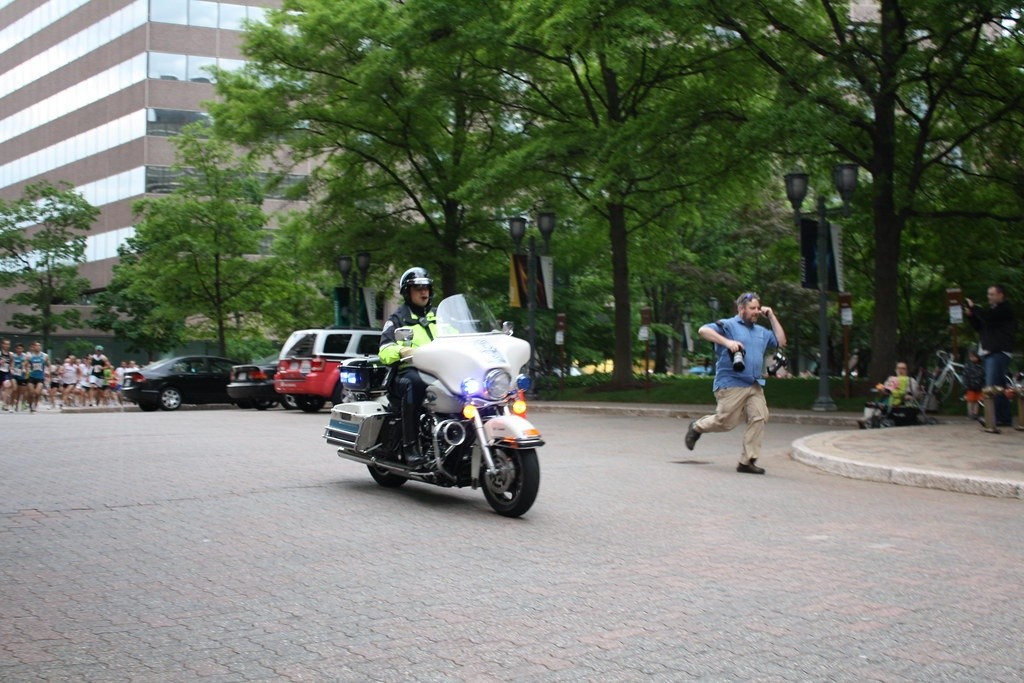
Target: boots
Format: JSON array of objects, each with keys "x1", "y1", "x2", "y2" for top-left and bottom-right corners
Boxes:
[{"x1": 403, "y1": 441, "x2": 428, "y2": 467}]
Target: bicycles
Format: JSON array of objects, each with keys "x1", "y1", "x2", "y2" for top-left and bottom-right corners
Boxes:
[{"x1": 917, "y1": 349, "x2": 1015, "y2": 415}]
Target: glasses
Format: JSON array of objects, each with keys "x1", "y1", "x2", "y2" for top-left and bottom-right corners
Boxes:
[
  {"x1": 740, "y1": 292, "x2": 758, "y2": 304},
  {"x1": 411, "y1": 285, "x2": 429, "y2": 290}
]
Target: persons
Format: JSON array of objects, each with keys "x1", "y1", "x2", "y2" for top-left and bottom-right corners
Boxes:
[
  {"x1": 379, "y1": 267, "x2": 460, "y2": 467},
  {"x1": 962, "y1": 284, "x2": 1013, "y2": 426},
  {"x1": 685, "y1": 291, "x2": 786, "y2": 474},
  {"x1": 0, "y1": 338, "x2": 139, "y2": 412},
  {"x1": 858, "y1": 362, "x2": 922, "y2": 429}
]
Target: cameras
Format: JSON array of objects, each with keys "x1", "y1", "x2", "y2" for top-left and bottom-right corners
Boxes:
[
  {"x1": 728, "y1": 346, "x2": 746, "y2": 373},
  {"x1": 766, "y1": 353, "x2": 788, "y2": 376}
]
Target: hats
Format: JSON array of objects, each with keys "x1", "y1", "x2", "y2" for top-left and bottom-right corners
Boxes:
[{"x1": 95, "y1": 345, "x2": 103, "y2": 351}]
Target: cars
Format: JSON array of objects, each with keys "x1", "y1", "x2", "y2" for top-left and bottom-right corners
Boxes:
[
  {"x1": 119, "y1": 354, "x2": 247, "y2": 412},
  {"x1": 225, "y1": 351, "x2": 300, "y2": 411}
]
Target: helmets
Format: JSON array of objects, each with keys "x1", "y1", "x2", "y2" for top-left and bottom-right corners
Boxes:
[{"x1": 400, "y1": 267, "x2": 433, "y2": 294}]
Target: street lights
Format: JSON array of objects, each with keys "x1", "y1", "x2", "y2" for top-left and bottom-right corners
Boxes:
[
  {"x1": 508, "y1": 211, "x2": 558, "y2": 402},
  {"x1": 333, "y1": 249, "x2": 374, "y2": 330},
  {"x1": 782, "y1": 162, "x2": 859, "y2": 412}
]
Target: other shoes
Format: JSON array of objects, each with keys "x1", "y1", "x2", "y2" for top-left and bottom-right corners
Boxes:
[
  {"x1": 685, "y1": 421, "x2": 701, "y2": 450},
  {"x1": 736, "y1": 458, "x2": 764, "y2": 474}
]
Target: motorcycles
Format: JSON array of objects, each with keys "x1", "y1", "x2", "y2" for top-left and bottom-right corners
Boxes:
[{"x1": 322, "y1": 295, "x2": 546, "y2": 518}]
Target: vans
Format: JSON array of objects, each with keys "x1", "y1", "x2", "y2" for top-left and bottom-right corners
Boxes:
[{"x1": 273, "y1": 328, "x2": 383, "y2": 413}]
